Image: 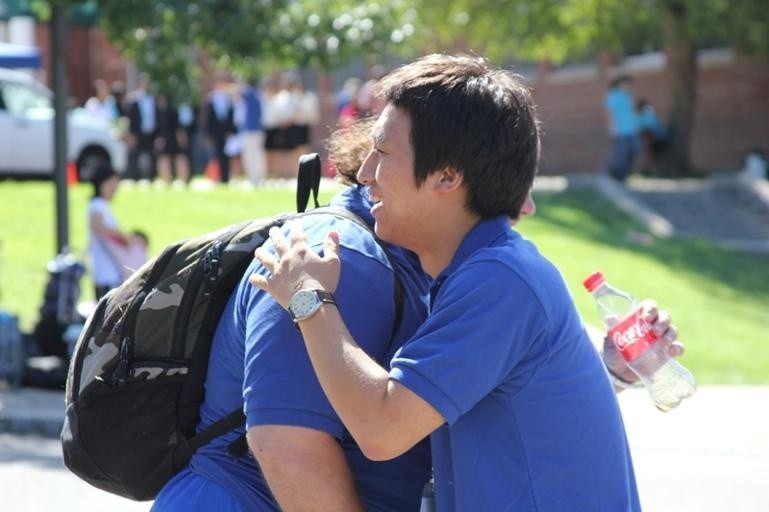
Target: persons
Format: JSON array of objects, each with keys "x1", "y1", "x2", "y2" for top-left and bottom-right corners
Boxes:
[
  {"x1": 324, "y1": 77, "x2": 378, "y2": 178},
  {"x1": 84, "y1": 72, "x2": 321, "y2": 192},
  {"x1": 244, "y1": 48, "x2": 643, "y2": 510},
  {"x1": 100, "y1": 225, "x2": 155, "y2": 293},
  {"x1": 84, "y1": 169, "x2": 127, "y2": 304},
  {"x1": 608, "y1": 75, "x2": 637, "y2": 177},
  {"x1": 633, "y1": 101, "x2": 664, "y2": 142},
  {"x1": 138, "y1": 44, "x2": 683, "y2": 512}
]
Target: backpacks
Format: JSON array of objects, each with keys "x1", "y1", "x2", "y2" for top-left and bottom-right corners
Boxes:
[{"x1": 57, "y1": 206, "x2": 401, "y2": 505}]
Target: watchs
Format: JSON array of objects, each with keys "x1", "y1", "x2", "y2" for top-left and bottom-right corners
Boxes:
[{"x1": 285, "y1": 286, "x2": 341, "y2": 328}]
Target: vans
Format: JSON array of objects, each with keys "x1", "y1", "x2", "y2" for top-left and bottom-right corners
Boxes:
[{"x1": 0, "y1": 66, "x2": 131, "y2": 185}]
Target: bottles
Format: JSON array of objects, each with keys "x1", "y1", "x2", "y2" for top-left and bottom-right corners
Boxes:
[{"x1": 584, "y1": 271, "x2": 697, "y2": 414}]
